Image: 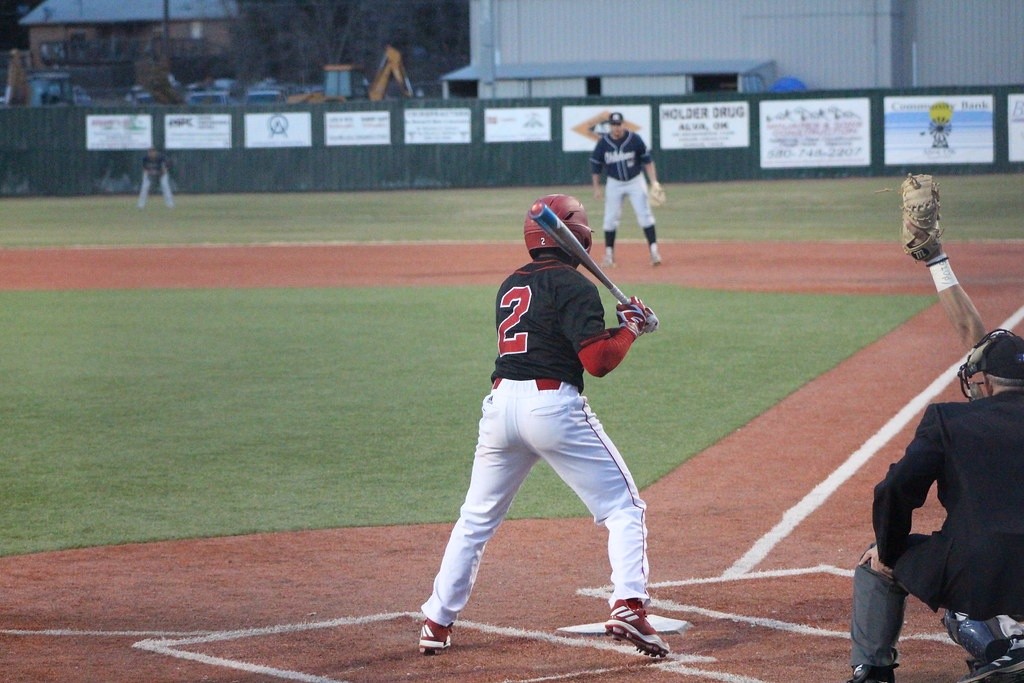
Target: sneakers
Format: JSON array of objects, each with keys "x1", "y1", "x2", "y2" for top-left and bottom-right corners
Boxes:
[
  {"x1": 604, "y1": 598, "x2": 670, "y2": 658},
  {"x1": 847, "y1": 664, "x2": 898, "y2": 683},
  {"x1": 419, "y1": 619, "x2": 455, "y2": 655},
  {"x1": 954, "y1": 646, "x2": 1024, "y2": 682}
]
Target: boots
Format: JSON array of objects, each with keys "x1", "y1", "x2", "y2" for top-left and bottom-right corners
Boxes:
[
  {"x1": 601, "y1": 246, "x2": 614, "y2": 267},
  {"x1": 650, "y1": 241, "x2": 661, "y2": 265}
]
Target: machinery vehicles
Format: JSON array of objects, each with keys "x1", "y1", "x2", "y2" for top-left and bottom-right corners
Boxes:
[
  {"x1": 132, "y1": 26, "x2": 194, "y2": 104},
  {"x1": 283, "y1": 45, "x2": 417, "y2": 104},
  {"x1": 5, "y1": 50, "x2": 76, "y2": 107}
]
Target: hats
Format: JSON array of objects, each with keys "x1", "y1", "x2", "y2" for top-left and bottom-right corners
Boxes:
[
  {"x1": 973, "y1": 336, "x2": 1024, "y2": 379},
  {"x1": 608, "y1": 112, "x2": 623, "y2": 124}
]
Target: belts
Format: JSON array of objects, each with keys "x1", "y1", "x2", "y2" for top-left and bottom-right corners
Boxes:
[{"x1": 492, "y1": 377, "x2": 564, "y2": 388}]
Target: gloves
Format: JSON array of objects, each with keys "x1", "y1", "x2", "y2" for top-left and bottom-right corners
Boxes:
[
  {"x1": 645, "y1": 307, "x2": 659, "y2": 334},
  {"x1": 615, "y1": 296, "x2": 647, "y2": 344}
]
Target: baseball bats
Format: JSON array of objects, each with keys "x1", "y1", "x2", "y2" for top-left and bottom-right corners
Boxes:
[{"x1": 528, "y1": 200, "x2": 633, "y2": 306}]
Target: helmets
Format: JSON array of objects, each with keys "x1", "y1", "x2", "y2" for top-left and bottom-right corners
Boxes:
[{"x1": 523, "y1": 194, "x2": 593, "y2": 260}]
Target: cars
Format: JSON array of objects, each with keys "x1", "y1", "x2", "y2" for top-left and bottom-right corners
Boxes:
[{"x1": 68, "y1": 52, "x2": 325, "y2": 106}]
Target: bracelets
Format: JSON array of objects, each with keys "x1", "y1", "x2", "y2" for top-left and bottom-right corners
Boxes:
[{"x1": 926, "y1": 257, "x2": 950, "y2": 268}]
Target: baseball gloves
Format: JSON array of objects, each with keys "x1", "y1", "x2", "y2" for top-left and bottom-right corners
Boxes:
[
  {"x1": 649, "y1": 184, "x2": 666, "y2": 207},
  {"x1": 899, "y1": 174, "x2": 944, "y2": 262}
]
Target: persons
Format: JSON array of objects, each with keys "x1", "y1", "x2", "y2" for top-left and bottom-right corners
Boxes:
[
  {"x1": 848, "y1": 331, "x2": 1024, "y2": 683},
  {"x1": 902, "y1": 173, "x2": 1024, "y2": 683},
  {"x1": 138, "y1": 148, "x2": 175, "y2": 214},
  {"x1": 589, "y1": 112, "x2": 666, "y2": 269},
  {"x1": 417, "y1": 196, "x2": 673, "y2": 660}
]
